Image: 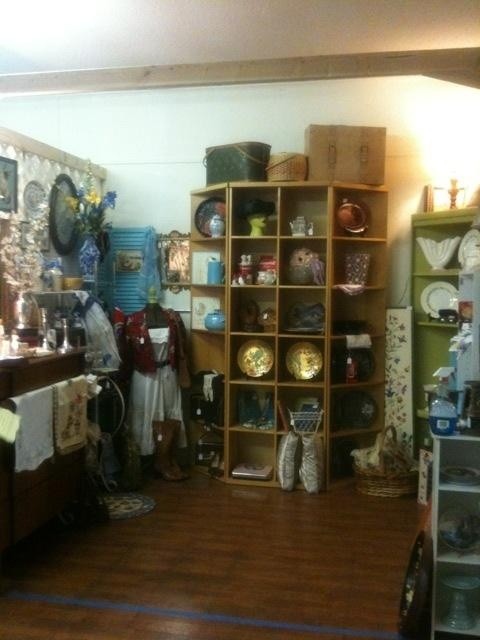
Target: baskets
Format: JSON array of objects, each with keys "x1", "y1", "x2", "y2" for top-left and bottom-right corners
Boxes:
[
  {"x1": 266, "y1": 151, "x2": 306, "y2": 182},
  {"x1": 350, "y1": 426, "x2": 419, "y2": 499},
  {"x1": 287, "y1": 407, "x2": 323, "y2": 436}
]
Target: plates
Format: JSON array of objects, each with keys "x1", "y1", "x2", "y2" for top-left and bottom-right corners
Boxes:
[
  {"x1": 237, "y1": 339, "x2": 324, "y2": 380},
  {"x1": 420, "y1": 283, "x2": 459, "y2": 320},
  {"x1": 458, "y1": 228, "x2": 480, "y2": 266},
  {"x1": 439, "y1": 465, "x2": 480, "y2": 551},
  {"x1": 195, "y1": 197, "x2": 225, "y2": 236},
  {"x1": 23, "y1": 179, "x2": 46, "y2": 212}
]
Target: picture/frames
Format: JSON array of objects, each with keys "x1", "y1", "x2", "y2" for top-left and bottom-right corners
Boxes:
[{"x1": 0, "y1": 158, "x2": 18, "y2": 213}]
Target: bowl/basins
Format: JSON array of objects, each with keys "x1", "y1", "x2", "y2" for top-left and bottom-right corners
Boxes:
[{"x1": 65, "y1": 278, "x2": 85, "y2": 290}]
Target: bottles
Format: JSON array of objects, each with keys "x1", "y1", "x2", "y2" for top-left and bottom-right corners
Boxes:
[
  {"x1": 204, "y1": 310, "x2": 225, "y2": 329},
  {"x1": 209, "y1": 213, "x2": 225, "y2": 238},
  {"x1": 41, "y1": 313, "x2": 75, "y2": 356}
]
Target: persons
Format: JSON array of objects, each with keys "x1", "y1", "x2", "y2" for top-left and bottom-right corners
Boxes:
[
  {"x1": 238, "y1": 199, "x2": 274, "y2": 236},
  {"x1": 124, "y1": 303, "x2": 188, "y2": 456}
]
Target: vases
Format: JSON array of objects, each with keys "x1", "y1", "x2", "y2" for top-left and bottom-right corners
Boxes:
[{"x1": 77, "y1": 231, "x2": 101, "y2": 284}]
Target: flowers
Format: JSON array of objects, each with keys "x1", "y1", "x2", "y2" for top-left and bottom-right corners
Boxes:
[{"x1": 50, "y1": 160, "x2": 118, "y2": 236}]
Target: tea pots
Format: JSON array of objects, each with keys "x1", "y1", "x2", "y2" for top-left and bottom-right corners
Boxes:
[{"x1": 289, "y1": 216, "x2": 314, "y2": 237}]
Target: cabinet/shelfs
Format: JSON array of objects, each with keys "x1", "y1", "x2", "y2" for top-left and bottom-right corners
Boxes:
[
  {"x1": 1, "y1": 342, "x2": 130, "y2": 559},
  {"x1": 406, "y1": 207, "x2": 480, "y2": 639},
  {"x1": 184, "y1": 180, "x2": 390, "y2": 495}
]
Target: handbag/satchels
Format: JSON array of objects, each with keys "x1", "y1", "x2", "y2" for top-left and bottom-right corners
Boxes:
[{"x1": 197, "y1": 431, "x2": 224, "y2": 466}]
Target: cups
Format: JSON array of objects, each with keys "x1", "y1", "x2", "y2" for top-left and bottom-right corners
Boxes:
[{"x1": 207, "y1": 261, "x2": 226, "y2": 284}]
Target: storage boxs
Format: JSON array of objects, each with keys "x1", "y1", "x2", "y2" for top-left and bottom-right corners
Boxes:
[{"x1": 304, "y1": 121, "x2": 385, "y2": 184}]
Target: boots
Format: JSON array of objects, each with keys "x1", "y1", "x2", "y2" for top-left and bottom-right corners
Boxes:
[
  {"x1": 150, "y1": 418, "x2": 183, "y2": 481},
  {"x1": 168, "y1": 414, "x2": 191, "y2": 480}
]
